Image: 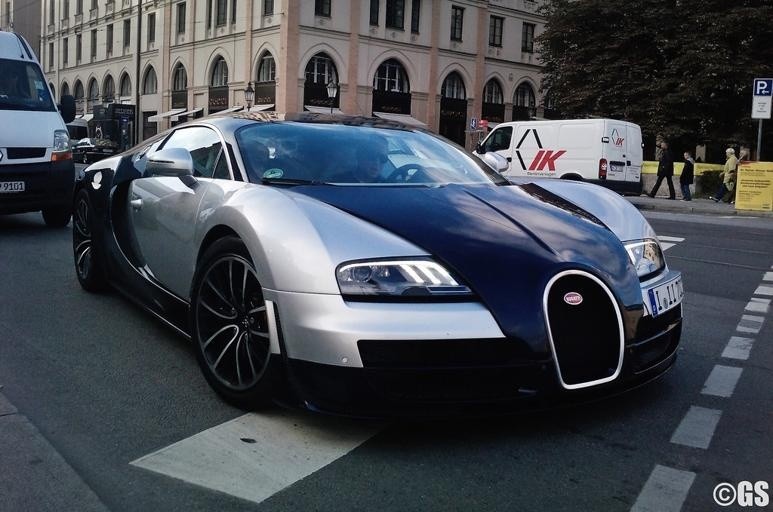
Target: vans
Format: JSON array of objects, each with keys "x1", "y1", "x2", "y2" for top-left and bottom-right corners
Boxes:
[
  {"x1": 469, "y1": 117, "x2": 647, "y2": 190},
  {"x1": 1, "y1": 26, "x2": 83, "y2": 225}
]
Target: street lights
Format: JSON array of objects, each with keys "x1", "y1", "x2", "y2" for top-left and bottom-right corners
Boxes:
[
  {"x1": 240, "y1": 80, "x2": 255, "y2": 114},
  {"x1": 323, "y1": 77, "x2": 339, "y2": 122}
]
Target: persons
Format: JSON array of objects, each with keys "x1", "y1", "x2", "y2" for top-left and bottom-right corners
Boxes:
[
  {"x1": 324, "y1": 135, "x2": 389, "y2": 183},
  {"x1": 709, "y1": 146, "x2": 750, "y2": 204},
  {"x1": 646, "y1": 140, "x2": 675, "y2": 200},
  {"x1": 679, "y1": 151, "x2": 695, "y2": 200}
]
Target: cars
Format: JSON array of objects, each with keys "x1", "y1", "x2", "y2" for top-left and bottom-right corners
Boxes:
[{"x1": 69, "y1": 133, "x2": 122, "y2": 160}]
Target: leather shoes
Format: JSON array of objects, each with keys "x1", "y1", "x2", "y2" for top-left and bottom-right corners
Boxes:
[{"x1": 646, "y1": 193, "x2": 675, "y2": 200}]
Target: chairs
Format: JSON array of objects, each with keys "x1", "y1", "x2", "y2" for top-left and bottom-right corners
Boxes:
[{"x1": 225, "y1": 139, "x2": 358, "y2": 180}]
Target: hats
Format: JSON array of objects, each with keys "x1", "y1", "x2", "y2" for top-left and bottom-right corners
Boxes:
[{"x1": 725, "y1": 148, "x2": 735, "y2": 153}]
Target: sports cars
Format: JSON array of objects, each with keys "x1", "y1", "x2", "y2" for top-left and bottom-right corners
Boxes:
[{"x1": 78, "y1": 110, "x2": 688, "y2": 419}]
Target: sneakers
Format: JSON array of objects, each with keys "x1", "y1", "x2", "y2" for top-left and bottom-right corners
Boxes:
[{"x1": 680, "y1": 196, "x2": 719, "y2": 202}]
